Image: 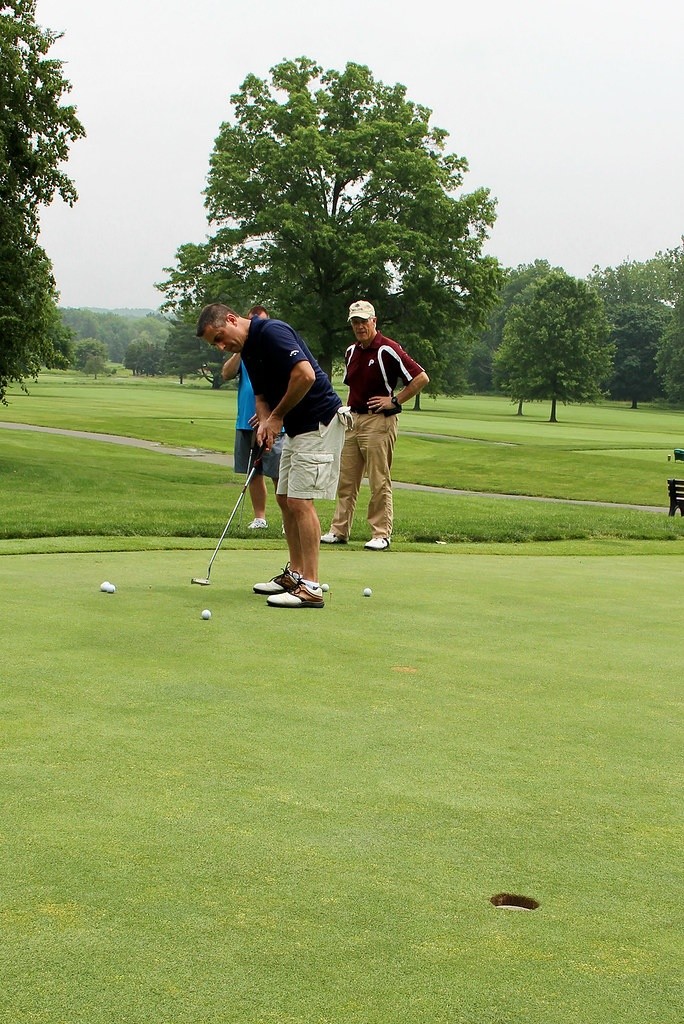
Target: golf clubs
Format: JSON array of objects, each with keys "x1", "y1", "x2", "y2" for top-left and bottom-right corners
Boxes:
[
  {"x1": 190, "y1": 439, "x2": 267, "y2": 587},
  {"x1": 236, "y1": 425, "x2": 257, "y2": 529}
]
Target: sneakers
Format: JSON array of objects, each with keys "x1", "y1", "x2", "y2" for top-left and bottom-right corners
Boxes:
[
  {"x1": 320, "y1": 533, "x2": 347, "y2": 544},
  {"x1": 266, "y1": 570, "x2": 325, "y2": 608},
  {"x1": 252, "y1": 562, "x2": 300, "y2": 594},
  {"x1": 247, "y1": 520, "x2": 269, "y2": 528},
  {"x1": 364, "y1": 536, "x2": 391, "y2": 551},
  {"x1": 282, "y1": 520, "x2": 286, "y2": 535}
]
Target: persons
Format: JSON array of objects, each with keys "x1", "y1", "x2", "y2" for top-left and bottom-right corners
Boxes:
[
  {"x1": 196, "y1": 304, "x2": 353, "y2": 608},
  {"x1": 320, "y1": 301, "x2": 429, "y2": 550},
  {"x1": 223, "y1": 306, "x2": 286, "y2": 534}
]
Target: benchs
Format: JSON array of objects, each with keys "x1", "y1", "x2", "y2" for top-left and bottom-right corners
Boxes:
[{"x1": 667, "y1": 480, "x2": 684, "y2": 517}]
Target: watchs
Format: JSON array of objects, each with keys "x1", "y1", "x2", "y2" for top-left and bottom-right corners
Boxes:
[{"x1": 391, "y1": 397, "x2": 399, "y2": 407}]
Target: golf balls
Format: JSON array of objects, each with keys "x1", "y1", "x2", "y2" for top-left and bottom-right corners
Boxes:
[
  {"x1": 201, "y1": 609, "x2": 211, "y2": 619},
  {"x1": 100, "y1": 581, "x2": 116, "y2": 593},
  {"x1": 363, "y1": 588, "x2": 372, "y2": 596},
  {"x1": 322, "y1": 583, "x2": 329, "y2": 592}
]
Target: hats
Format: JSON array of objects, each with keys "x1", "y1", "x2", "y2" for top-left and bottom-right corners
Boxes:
[{"x1": 347, "y1": 300, "x2": 376, "y2": 323}]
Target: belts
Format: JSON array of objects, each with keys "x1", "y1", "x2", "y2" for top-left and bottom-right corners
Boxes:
[{"x1": 350, "y1": 406, "x2": 382, "y2": 415}]
